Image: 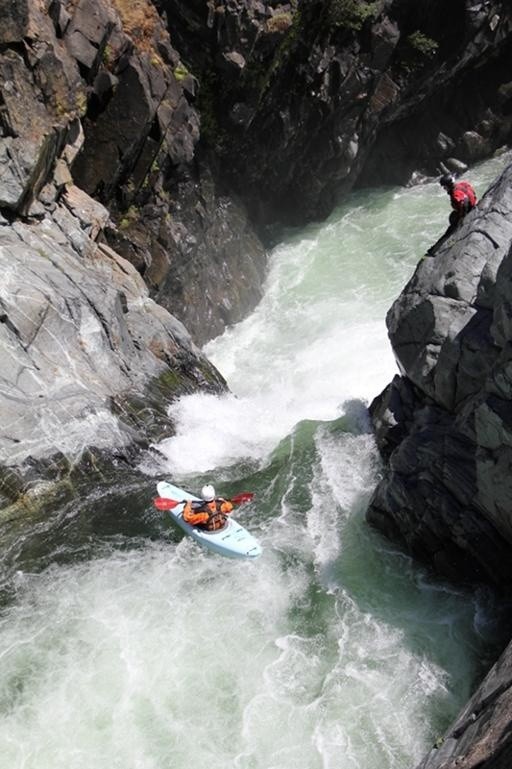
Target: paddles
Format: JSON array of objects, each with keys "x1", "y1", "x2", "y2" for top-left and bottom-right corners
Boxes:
[{"x1": 154, "y1": 493, "x2": 253, "y2": 511}]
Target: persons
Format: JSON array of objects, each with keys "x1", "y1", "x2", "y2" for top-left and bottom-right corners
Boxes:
[
  {"x1": 439, "y1": 174, "x2": 476, "y2": 226},
  {"x1": 183, "y1": 485, "x2": 232, "y2": 532}
]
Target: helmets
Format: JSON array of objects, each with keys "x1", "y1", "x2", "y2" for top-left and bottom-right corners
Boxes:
[
  {"x1": 200, "y1": 484, "x2": 215, "y2": 501},
  {"x1": 440, "y1": 174, "x2": 455, "y2": 188}
]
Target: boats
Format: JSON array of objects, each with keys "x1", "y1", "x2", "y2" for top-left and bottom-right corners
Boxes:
[{"x1": 155, "y1": 481, "x2": 264, "y2": 560}]
